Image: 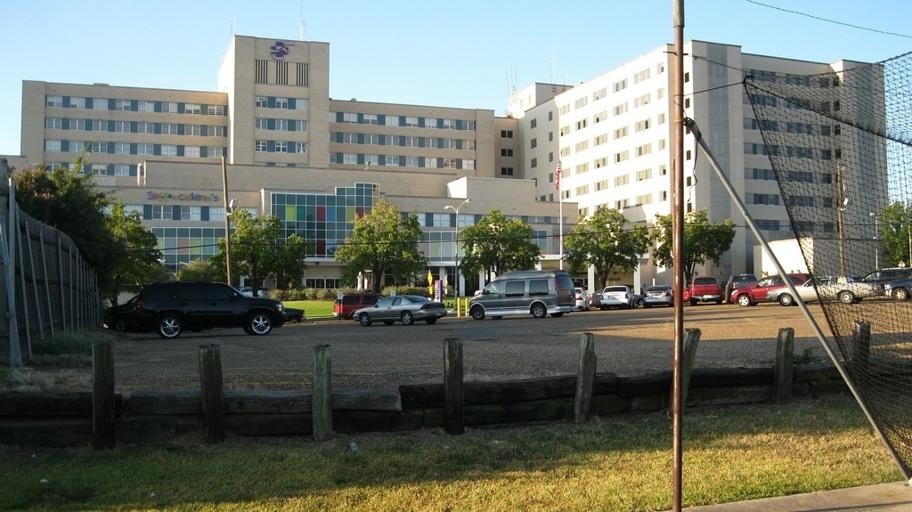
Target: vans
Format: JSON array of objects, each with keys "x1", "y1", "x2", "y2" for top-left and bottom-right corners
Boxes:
[{"x1": 467, "y1": 269, "x2": 577, "y2": 321}]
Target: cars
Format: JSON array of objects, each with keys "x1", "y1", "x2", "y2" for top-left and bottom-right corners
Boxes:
[
  {"x1": 724, "y1": 272, "x2": 815, "y2": 307},
  {"x1": 332, "y1": 291, "x2": 387, "y2": 321},
  {"x1": 351, "y1": 294, "x2": 449, "y2": 329},
  {"x1": 689, "y1": 274, "x2": 723, "y2": 307},
  {"x1": 102, "y1": 292, "x2": 156, "y2": 335},
  {"x1": 641, "y1": 284, "x2": 675, "y2": 308},
  {"x1": 600, "y1": 284, "x2": 636, "y2": 311},
  {"x1": 570, "y1": 286, "x2": 592, "y2": 313},
  {"x1": 859, "y1": 266, "x2": 912, "y2": 302}
]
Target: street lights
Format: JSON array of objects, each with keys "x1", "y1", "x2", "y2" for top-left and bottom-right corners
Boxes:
[
  {"x1": 441, "y1": 197, "x2": 478, "y2": 319},
  {"x1": 217, "y1": 153, "x2": 240, "y2": 291}
]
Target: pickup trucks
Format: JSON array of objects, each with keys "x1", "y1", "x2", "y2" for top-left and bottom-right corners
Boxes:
[{"x1": 766, "y1": 267, "x2": 883, "y2": 309}]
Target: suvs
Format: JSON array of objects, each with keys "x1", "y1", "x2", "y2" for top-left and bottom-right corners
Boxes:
[{"x1": 134, "y1": 281, "x2": 287, "y2": 340}]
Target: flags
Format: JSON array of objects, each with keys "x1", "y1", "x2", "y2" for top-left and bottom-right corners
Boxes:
[{"x1": 555, "y1": 162, "x2": 561, "y2": 190}]
